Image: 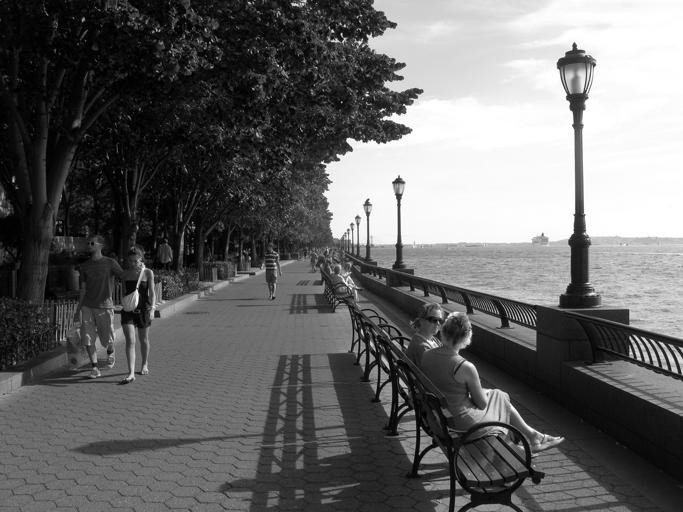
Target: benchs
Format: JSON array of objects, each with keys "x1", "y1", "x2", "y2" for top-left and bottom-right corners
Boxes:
[
  {"x1": 348, "y1": 299, "x2": 544, "y2": 512},
  {"x1": 319, "y1": 262, "x2": 358, "y2": 313}
]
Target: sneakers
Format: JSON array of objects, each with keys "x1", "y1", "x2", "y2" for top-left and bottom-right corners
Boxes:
[
  {"x1": 106, "y1": 348, "x2": 115, "y2": 369},
  {"x1": 89, "y1": 367, "x2": 102, "y2": 379}
]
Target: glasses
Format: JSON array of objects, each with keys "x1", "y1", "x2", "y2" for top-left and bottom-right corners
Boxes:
[
  {"x1": 87, "y1": 240, "x2": 99, "y2": 247},
  {"x1": 423, "y1": 316, "x2": 444, "y2": 324}
]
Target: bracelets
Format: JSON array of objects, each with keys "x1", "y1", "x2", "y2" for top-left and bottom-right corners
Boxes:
[{"x1": 150, "y1": 306, "x2": 156, "y2": 309}]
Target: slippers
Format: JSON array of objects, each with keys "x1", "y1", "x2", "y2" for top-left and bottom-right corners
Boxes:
[{"x1": 532, "y1": 434, "x2": 565, "y2": 453}]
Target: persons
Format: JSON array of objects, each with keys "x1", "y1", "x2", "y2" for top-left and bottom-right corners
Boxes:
[
  {"x1": 156, "y1": 239, "x2": 173, "y2": 272},
  {"x1": 261, "y1": 243, "x2": 282, "y2": 300},
  {"x1": 74, "y1": 234, "x2": 124, "y2": 379},
  {"x1": 121, "y1": 246, "x2": 156, "y2": 384},
  {"x1": 303, "y1": 246, "x2": 365, "y2": 308},
  {"x1": 407, "y1": 302, "x2": 445, "y2": 369},
  {"x1": 422, "y1": 311, "x2": 566, "y2": 458}
]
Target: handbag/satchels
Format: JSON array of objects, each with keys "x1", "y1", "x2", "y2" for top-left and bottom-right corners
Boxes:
[{"x1": 122, "y1": 289, "x2": 140, "y2": 312}]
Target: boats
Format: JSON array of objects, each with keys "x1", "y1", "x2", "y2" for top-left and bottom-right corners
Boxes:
[{"x1": 532, "y1": 233, "x2": 549, "y2": 244}]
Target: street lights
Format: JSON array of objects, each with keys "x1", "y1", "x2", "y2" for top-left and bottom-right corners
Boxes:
[
  {"x1": 335, "y1": 199, "x2": 372, "y2": 259},
  {"x1": 555, "y1": 43, "x2": 596, "y2": 293},
  {"x1": 393, "y1": 176, "x2": 406, "y2": 268}
]
